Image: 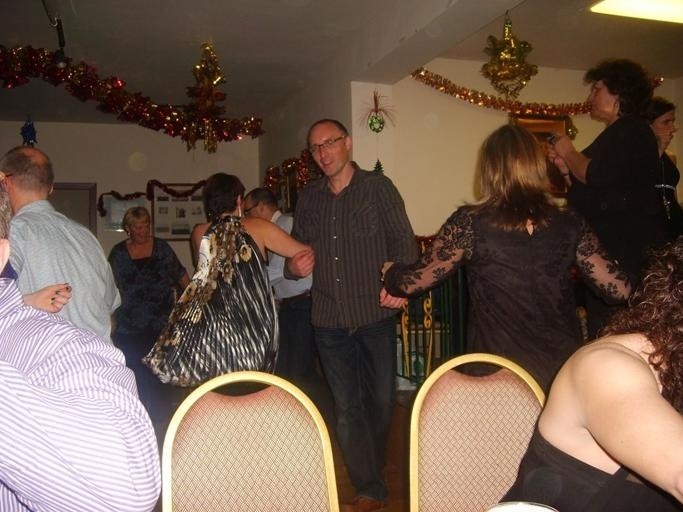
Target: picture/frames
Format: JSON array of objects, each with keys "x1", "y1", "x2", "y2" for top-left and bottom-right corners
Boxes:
[
  {"x1": 508, "y1": 113, "x2": 575, "y2": 199},
  {"x1": 273, "y1": 168, "x2": 302, "y2": 216}
]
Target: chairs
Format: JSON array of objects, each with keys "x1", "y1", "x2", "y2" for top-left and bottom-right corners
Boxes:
[
  {"x1": 408, "y1": 351, "x2": 549, "y2": 512},
  {"x1": 161, "y1": 370, "x2": 340, "y2": 512}
]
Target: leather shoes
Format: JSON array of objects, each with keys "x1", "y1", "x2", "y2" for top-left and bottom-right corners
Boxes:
[{"x1": 341, "y1": 493, "x2": 387, "y2": 512}]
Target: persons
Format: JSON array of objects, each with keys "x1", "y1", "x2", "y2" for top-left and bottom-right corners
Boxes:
[
  {"x1": 381, "y1": 126, "x2": 633, "y2": 393},
  {"x1": 107, "y1": 206, "x2": 189, "y2": 356},
  {"x1": 0, "y1": 175, "x2": 161, "y2": 512},
  {"x1": 285, "y1": 118, "x2": 420, "y2": 512},
  {"x1": 25, "y1": 277, "x2": 70, "y2": 313},
  {"x1": 0, "y1": 146, "x2": 122, "y2": 360},
  {"x1": 489, "y1": 233, "x2": 683, "y2": 510},
  {"x1": 549, "y1": 59, "x2": 657, "y2": 277},
  {"x1": 245, "y1": 187, "x2": 312, "y2": 375},
  {"x1": 141, "y1": 172, "x2": 311, "y2": 388},
  {"x1": 651, "y1": 97, "x2": 683, "y2": 230}
]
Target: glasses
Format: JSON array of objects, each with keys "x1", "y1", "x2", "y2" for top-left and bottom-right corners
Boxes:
[{"x1": 308, "y1": 134, "x2": 346, "y2": 154}]
